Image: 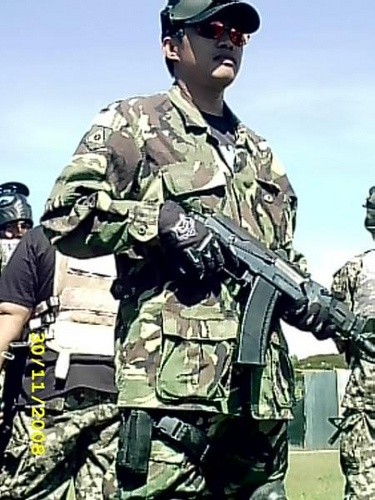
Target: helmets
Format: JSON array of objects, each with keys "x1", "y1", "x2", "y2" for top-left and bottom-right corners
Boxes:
[{"x1": 0, "y1": 192, "x2": 34, "y2": 228}]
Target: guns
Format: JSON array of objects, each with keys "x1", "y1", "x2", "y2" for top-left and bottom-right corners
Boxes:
[{"x1": 110, "y1": 210, "x2": 375, "y2": 367}]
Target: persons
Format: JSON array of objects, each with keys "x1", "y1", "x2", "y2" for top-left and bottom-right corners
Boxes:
[
  {"x1": 0, "y1": 180, "x2": 35, "y2": 270},
  {"x1": 40, "y1": 0, "x2": 344, "y2": 499},
  {"x1": 1, "y1": 226, "x2": 126, "y2": 500},
  {"x1": 334, "y1": 186, "x2": 375, "y2": 499}
]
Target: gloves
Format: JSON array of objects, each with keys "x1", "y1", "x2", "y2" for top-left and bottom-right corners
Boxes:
[
  {"x1": 281, "y1": 291, "x2": 347, "y2": 341},
  {"x1": 158, "y1": 201, "x2": 240, "y2": 286}
]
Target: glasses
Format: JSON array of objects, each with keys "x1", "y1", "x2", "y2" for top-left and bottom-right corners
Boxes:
[{"x1": 196, "y1": 19, "x2": 250, "y2": 46}]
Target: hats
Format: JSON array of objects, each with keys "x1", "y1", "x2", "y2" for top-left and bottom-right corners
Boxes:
[
  {"x1": 159, "y1": 0, "x2": 261, "y2": 44},
  {"x1": 362, "y1": 186, "x2": 375, "y2": 233}
]
